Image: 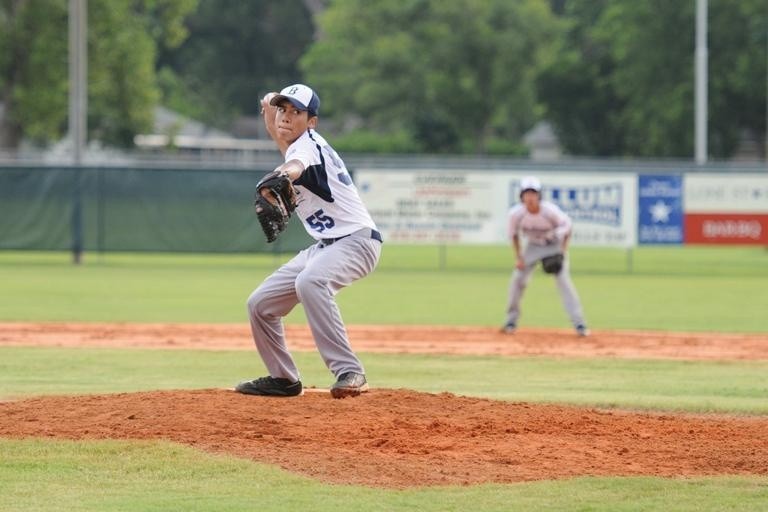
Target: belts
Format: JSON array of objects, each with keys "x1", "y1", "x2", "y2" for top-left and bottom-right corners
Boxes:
[{"x1": 322, "y1": 227, "x2": 384, "y2": 247}]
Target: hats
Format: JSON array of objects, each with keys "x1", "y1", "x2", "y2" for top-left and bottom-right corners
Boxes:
[
  {"x1": 270, "y1": 83, "x2": 320, "y2": 116},
  {"x1": 519, "y1": 176, "x2": 543, "y2": 198}
]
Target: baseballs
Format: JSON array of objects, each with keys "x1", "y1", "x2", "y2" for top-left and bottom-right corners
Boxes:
[{"x1": 263, "y1": 91, "x2": 280, "y2": 108}]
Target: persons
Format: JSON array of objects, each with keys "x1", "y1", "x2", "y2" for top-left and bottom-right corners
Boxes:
[
  {"x1": 235, "y1": 84, "x2": 382, "y2": 400},
  {"x1": 502, "y1": 177, "x2": 589, "y2": 334}
]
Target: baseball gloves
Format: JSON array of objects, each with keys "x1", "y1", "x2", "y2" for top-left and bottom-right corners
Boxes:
[
  {"x1": 255, "y1": 171, "x2": 300, "y2": 244},
  {"x1": 542, "y1": 253, "x2": 563, "y2": 274}
]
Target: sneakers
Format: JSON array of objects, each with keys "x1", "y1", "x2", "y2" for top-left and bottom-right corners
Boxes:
[
  {"x1": 501, "y1": 322, "x2": 590, "y2": 337},
  {"x1": 235, "y1": 375, "x2": 302, "y2": 397},
  {"x1": 331, "y1": 372, "x2": 371, "y2": 398}
]
